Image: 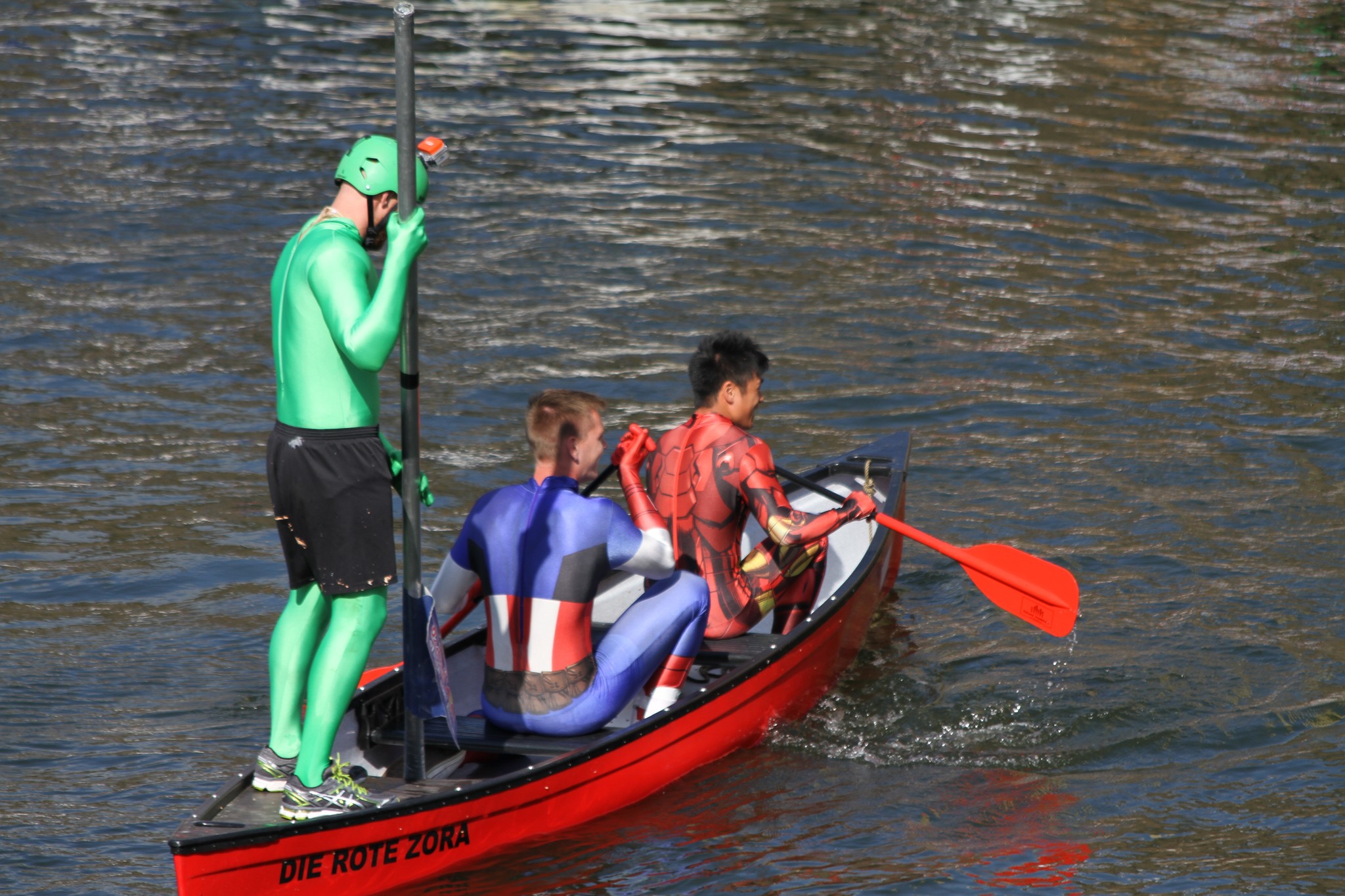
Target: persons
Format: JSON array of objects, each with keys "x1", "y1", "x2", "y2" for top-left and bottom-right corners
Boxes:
[
  {"x1": 429, "y1": 387, "x2": 711, "y2": 735},
  {"x1": 648, "y1": 333, "x2": 878, "y2": 681},
  {"x1": 252, "y1": 134, "x2": 434, "y2": 822}
]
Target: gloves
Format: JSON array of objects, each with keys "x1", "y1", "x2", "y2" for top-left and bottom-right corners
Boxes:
[
  {"x1": 836, "y1": 491, "x2": 878, "y2": 526},
  {"x1": 385, "y1": 208, "x2": 429, "y2": 261},
  {"x1": 610, "y1": 423, "x2": 656, "y2": 479},
  {"x1": 386, "y1": 450, "x2": 436, "y2": 508}
]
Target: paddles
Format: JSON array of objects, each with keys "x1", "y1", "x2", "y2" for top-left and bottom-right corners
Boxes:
[
  {"x1": 356, "y1": 424, "x2": 654, "y2": 693},
  {"x1": 770, "y1": 465, "x2": 1078, "y2": 642}
]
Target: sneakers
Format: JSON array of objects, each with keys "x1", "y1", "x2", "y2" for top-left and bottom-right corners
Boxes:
[
  {"x1": 277, "y1": 752, "x2": 400, "y2": 821},
  {"x1": 251, "y1": 745, "x2": 368, "y2": 792}
]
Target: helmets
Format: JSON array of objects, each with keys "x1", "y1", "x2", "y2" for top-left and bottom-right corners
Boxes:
[{"x1": 333, "y1": 134, "x2": 430, "y2": 211}]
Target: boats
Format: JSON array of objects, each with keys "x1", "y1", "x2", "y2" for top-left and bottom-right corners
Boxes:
[{"x1": 168, "y1": 424, "x2": 919, "y2": 896}]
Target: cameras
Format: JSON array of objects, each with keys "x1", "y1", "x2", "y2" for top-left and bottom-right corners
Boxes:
[{"x1": 418, "y1": 136, "x2": 450, "y2": 168}]
